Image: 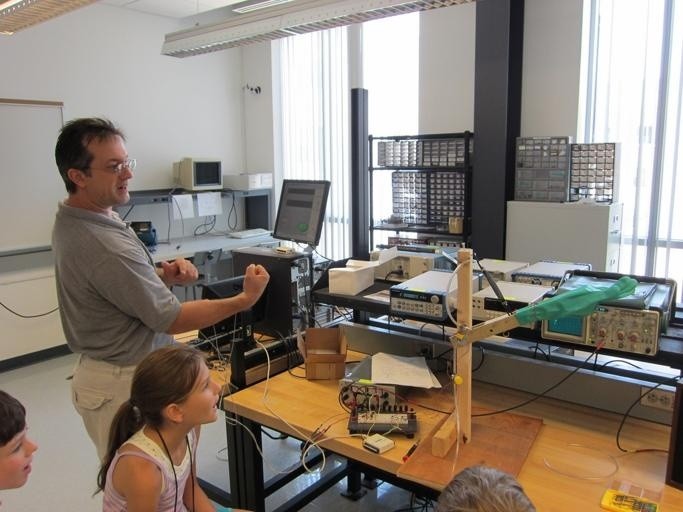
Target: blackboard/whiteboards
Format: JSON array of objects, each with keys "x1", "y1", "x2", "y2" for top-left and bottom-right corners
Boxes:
[{"x1": 0, "y1": 98, "x2": 70, "y2": 256}]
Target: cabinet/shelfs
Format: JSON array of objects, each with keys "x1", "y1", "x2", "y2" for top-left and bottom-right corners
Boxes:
[
  {"x1": 365, "y1": 130, "x2": 472, "y2": 252},
  {"x1": 505, "y1": 201, "x2": 623, "y2": 274}
]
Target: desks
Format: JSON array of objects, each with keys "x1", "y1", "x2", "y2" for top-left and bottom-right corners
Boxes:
[{"x1": 144, "y1": 228, "x2": 280, "y2": 267}]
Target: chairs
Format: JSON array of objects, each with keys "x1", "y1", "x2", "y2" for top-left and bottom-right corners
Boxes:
[{"x1": 169, "y1": 248, "x2": 222, "y2": 301}]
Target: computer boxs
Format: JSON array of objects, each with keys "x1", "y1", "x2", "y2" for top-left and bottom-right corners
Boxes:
[
  {"x1": 224, "y1": 173, "x2": 273, "y2": 191},
  {"x1": 231, "y1": 246, "x2": 316, "y2": 338}
]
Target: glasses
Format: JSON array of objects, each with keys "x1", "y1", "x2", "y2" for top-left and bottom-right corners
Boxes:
[{"x1": 83, "y1": 158, "x2": 139, "y2": 174}]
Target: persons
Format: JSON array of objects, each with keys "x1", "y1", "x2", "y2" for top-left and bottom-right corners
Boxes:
[
  {"x1": 89, "y1": 342, "x2": 256, "y2": 511},
  {"x1": 0, "y1": 391, "x2": 37, "y2": 490},
  {"x1": 50, "y1": 115, "x2": 272, "y2": 466},
  {"x1": 433, "y1": 463, "x2": 537, "y2": 511}
]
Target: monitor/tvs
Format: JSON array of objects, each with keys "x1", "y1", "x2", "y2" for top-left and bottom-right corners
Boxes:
[
  {"x1": 272, "y1": 178, "x2": 331, "y2": 246},
  {"x1": 197, "y1": 274, "x2": 269, "y2": 352},
  {"x1": 173, "y1": 156, "x2": 224, "y2": 191}
]
